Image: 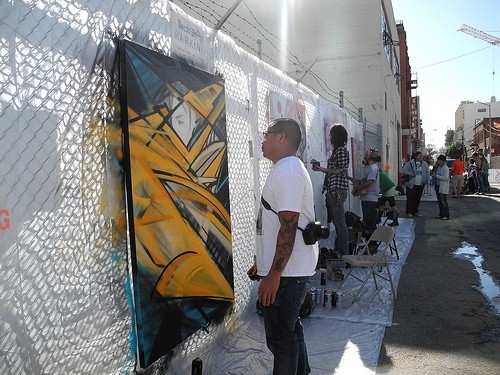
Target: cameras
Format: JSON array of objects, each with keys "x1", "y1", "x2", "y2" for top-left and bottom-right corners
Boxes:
[{"x1": 303, "y1": 222, "x2": 329, "y2": 245}]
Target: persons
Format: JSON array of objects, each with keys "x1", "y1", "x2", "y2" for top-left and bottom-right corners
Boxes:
[
  {"x1": 417, "y1": 147, "x2": 491, "y2": 220},
  {"x1": 248, "y1": 117, "x2": 322, "y2": 375},
  {"x1": 376, "y1": 169, "x2": 400, "y2": 227},
  {"x1": 309, "y1": 123, "x2": 351, "y2": 268},
  {"x1": 400, "y1": 152, "x2": 431, "y2": 217},
  {"x1": 345, "y1": 147, "x2": 381, "y2": 258}
]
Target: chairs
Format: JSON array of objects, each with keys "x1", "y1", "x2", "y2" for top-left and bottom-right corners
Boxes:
[
  {"x1": 337, "y1": 225, "x2": 395, "y2": 306},
  {"x1": 371, "y1": 210, "x2": 400, "y2": 260}
]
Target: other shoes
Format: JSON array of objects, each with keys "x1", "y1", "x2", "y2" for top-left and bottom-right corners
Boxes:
[
  {"x1": 434, "y1": 216, "x2": 441, "y2": 219},
  {"x1": 414, "y1": 213, "x2": 423, "y2": 217},
  {"x1": 441, "y1": 217, "x2": 449, "y2": 220},
  {"x1": 408, "y1": 212, "x2": 412, "y2": 217}
]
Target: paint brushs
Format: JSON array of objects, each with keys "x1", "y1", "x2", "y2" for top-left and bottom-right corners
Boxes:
[{"x1": 304, "y1": 161, "x2": 326, "y2": 163}]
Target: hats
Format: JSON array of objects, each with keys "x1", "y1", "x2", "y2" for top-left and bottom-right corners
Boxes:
[{"x1": 437, "y1": 154, "x2": 447, "y2": 161}]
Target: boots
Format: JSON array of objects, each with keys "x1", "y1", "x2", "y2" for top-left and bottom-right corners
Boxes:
[
  {"x1": 377, "y1": 209, "x2": 383, "y2": 222},
  {"x1": 388, "y1": 210, "x2": 399, "y2": 226}
]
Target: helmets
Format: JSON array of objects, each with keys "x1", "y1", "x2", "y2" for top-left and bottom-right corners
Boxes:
[{"x1": 365, "y1": 146, "x2": 379, "y2": 157}]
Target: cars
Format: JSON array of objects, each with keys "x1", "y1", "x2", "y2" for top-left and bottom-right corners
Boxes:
[{"x1": 445, "y1": 158, "x2": 468, "y2": 183}]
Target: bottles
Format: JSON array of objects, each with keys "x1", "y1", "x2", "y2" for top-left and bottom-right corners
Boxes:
[
  {"x1": 192, "y1": 357, "x2": 202, "y2": 375},
  {"x1": 310, "y1": 287, "x2": 342, "y2": 309}
]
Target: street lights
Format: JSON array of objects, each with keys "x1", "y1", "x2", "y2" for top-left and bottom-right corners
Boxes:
[{"x1": 477, "y1": 100, "x2": 492, "y2": 169}]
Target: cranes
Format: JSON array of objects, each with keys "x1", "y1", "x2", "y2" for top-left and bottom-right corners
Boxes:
[{"x1": 457, "y1": 24, "x2": 500, "y2": 47}]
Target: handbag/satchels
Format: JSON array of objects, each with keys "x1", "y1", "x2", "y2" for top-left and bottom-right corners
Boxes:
[{"x1": 401, "y1": 162, "x2": 415, "y2": 183}]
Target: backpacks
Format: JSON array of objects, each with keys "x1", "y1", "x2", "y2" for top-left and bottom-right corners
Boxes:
[
  {"x1": 338, "y1": 211, "x2": 379, "y2": 259},
  {"x1": 256, "y1": 284, "x2": 315, "y2": 319},
  {"x1": 316, "y1": 247, "x2": 350, "y2": 270}
]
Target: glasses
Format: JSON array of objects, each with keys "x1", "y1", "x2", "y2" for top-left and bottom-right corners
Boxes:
[{"x1": 263, "y1": 131, "x2": 287, "y2": 138}]
